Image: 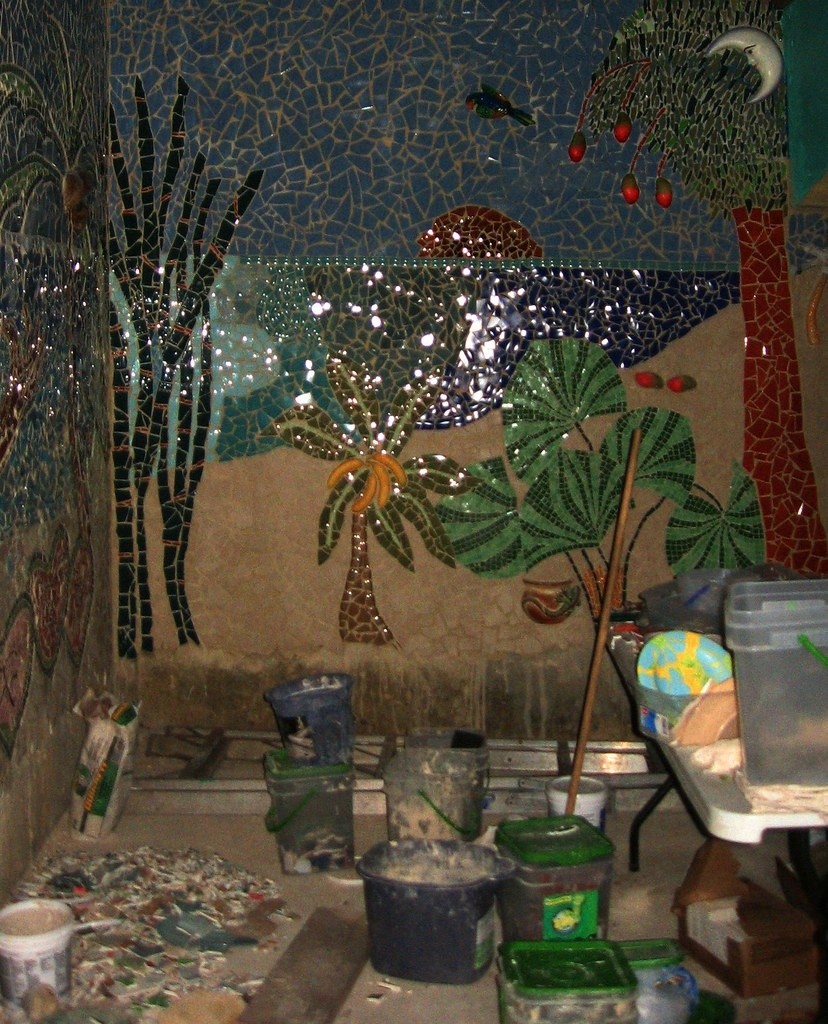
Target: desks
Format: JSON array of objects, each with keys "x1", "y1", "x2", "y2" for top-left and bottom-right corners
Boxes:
[{"x1": 604, "y1": 618, "x2": 828, "y2": 1024}]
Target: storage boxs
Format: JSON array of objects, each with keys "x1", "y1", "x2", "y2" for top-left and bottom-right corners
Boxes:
[{"x1": 673, "y1": 840, "x2": 820, "y2": 998}]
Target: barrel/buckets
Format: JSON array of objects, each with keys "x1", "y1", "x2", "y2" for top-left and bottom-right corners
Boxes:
[
  {"x1": 379, "y1": 724, "x2": 491, "y2": 841},
  {"x1": 262, "y1": 672, "x2": 356, "y2": 752},
  {"x1": 676, "y1": 568, "x2": 762, "y2": 614},
  {"x1": 496, "y1": 938, "x2": 639, "y2": 1024},
  {"x1": 495, "y1": 812, "x2": 616, "y2": 939},
  {"x1": 615, "y1": 938, "x2": 697, "y2": 1024},
  {"x1": 0, "y1": 898, "x2": 75, "y2": 1008},
  {"x1": 545, "y1": 774, "x2": 609, "y2": 828},
  {"x1": 260, "y1": 750, "x2": 356, "y2": 874},
  {"x1": 354, "y1": 837, "x2": 516, "y2": 986},
  {"x1": 722, "y1": 577, "x2": 828, "y2": 786}
]
